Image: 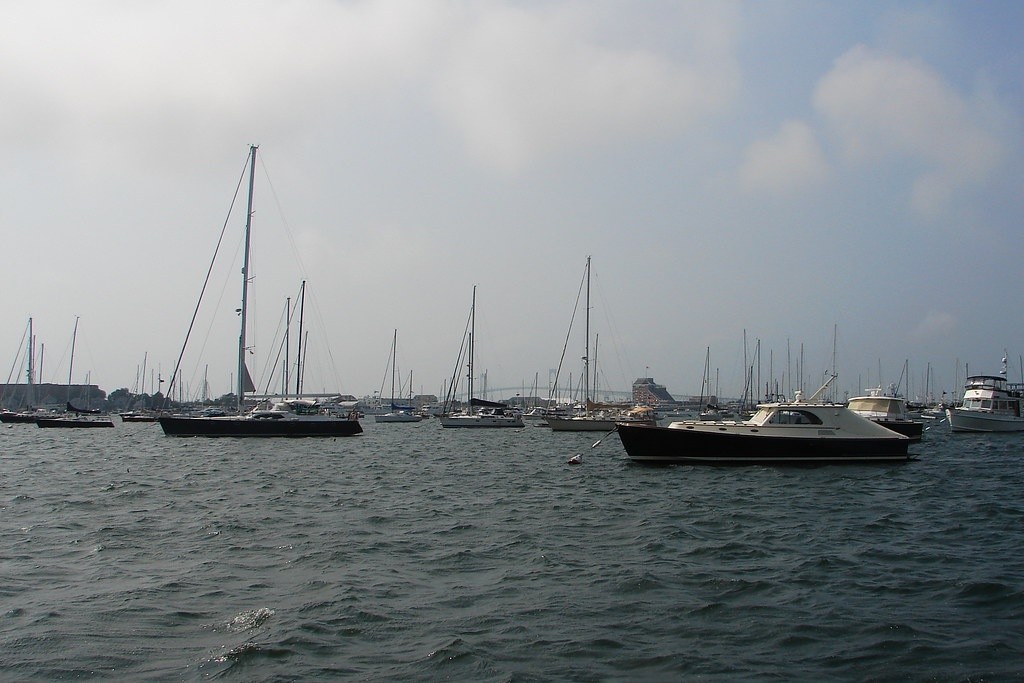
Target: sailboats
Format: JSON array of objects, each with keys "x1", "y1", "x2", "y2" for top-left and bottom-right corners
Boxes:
[
  {"x1": 33, "y1": 316, "x2": 116, "y2": 428},
  {"x1": 0, "y1": 314, "x2": 973, "y2": 421},
  {"x1": 371, "y1": 329, "x2": 425, "y2": 422},
  {"x1": 542, "y1": 253, "x2": 660, "y2": 432},
  {"x1": 155, "y1": 144, "x2": 366, "y2": 439},
  {"x1": 439, "y1": 284, "x2": 525, "y2": 428}
]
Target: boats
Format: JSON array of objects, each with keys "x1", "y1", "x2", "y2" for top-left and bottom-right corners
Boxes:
[
  {"x1": 613, "y1": 399, "x2": 914, "y2": 465},
  {"x1": 841, "y1": 394, "x2": 924, "y2": 443},
  {"x1": 946, "y1": 374, "x2": 1024, "y2": 433}
]
